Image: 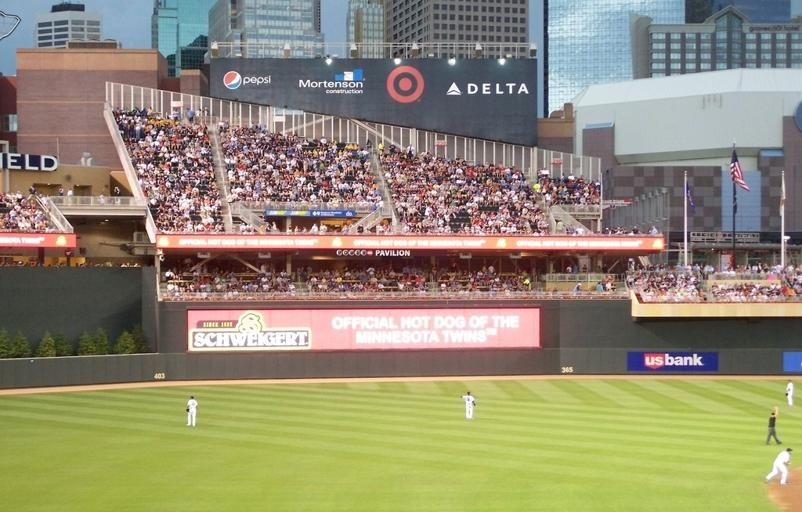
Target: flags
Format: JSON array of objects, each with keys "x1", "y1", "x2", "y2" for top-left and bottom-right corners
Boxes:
[
  {"x1": 779, "y1": 174, "x2": 786, "y2": 217},
  {"x1": 729, "y1": 149, "x2": 750, "y2": 192},
  {"x1": 687, "y1": 179, "x2": 696, "y2": 209}
]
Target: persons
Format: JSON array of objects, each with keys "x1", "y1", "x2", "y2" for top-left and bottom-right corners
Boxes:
[
  {"x1": 624, "y1": 260, "x2": 801, "y2": 302},
  {"x1": 765, "y1": 407, "x2": 782, "y2": 446},
  {"x1": 766, "y1": 447, "x2": 793, "y2": 485},
  {"x1": 566, "y1": 265, "x2": 572, "y2": 274},
  {"x1": 583, "y1": 264, "x2": 589, "y2": 272},
  {"x1": 461, "y1": 391, "x2": 476, "y2": 419},
  {"x1": 1, "y1": 256, "x2": 141, "y2": 268},
  {"x1": 58, "y1": 186, "x2": 64, "y2": 196},
  {"x1": 0, "y1": 184, "x2": 58, "y2": 234},
  {"x1": 595, "y1": 263, "x2": 617, "y2": 292},
  {"x1": 67, "y1": 188, "x2": 73, "y2": 196},
  {"x1": 573, "y1": 282, "x2": 583, "y2": 295},
  {"x1": 785, "y1": 379, "x2": 794, "y2": 405},
  {"x1": 99, "y1": 193, "x2": 105, "y2": 205},
  {"x1": 185, "y1": 395, "x2": 199, "y2": 428}
]
